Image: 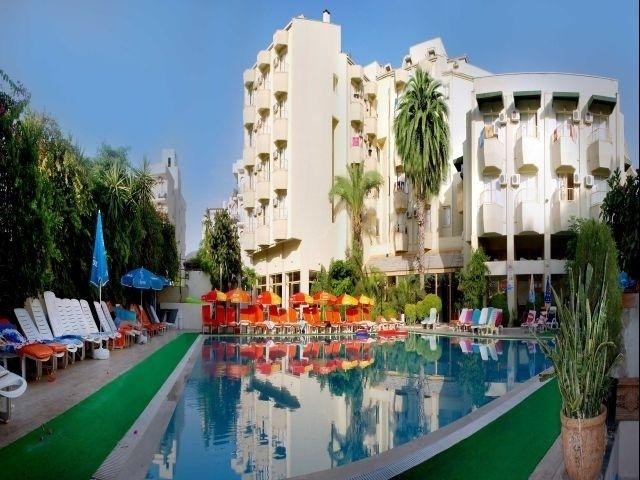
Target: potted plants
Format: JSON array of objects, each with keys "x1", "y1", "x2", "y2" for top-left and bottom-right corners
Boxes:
[
  {"x1": 567, "y1": 220, "x2": 623, "y2": 431},
  {"x1": 529, "y1": 263, "x2": 624, "y2": 480}
]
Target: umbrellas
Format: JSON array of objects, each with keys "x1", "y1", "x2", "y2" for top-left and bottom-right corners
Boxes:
[
  {"x1": 544, "y1": 275, "x2": 552, "y2": 319},
  {"x1": 120, "y1": 265, "x2": 165, "y2": 325},
  {"x1": 202, "y1": 342, "x2": 376, "y2": 376},
  {"x1": 152, "y1": 272, "x2": 174, "y2": 315},
  {"x1": 529, "y1": 274, "x2": 537, "y2": 311},
  {"x1": 198, "y1": 288, "x2": 374, "y2": 323},
  {"x1": 89, "y1": 209, "x2": 110, "y2": 350}
]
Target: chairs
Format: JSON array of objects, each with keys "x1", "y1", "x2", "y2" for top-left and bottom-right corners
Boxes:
[
  {"x1": 520, "y1": 307, "x2": 559, "y2": 335},
  {"x1": 202, "y1": 304, "x2": 373, "y2": 336},
  {"x1": 0, "y1": 290, "x2": 174, "y2": 423},
  {"x1": 376, "y1": 306, "x2": 504, "y2": 336}
]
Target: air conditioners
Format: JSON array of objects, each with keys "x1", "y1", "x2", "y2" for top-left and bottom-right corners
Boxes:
[
  {"x1": 573, "y1": 110, "x2": 580, "y2": 122},
  {"x1": 573, "y1": 174, "x2": 582, "y2": 185},
  {"x1": 498, "y1": 173, "x2": 507, "y2": 184},
  {"x1": 252, "y1": 58, "x2": 279, "y2": 218},
  {"x1": 512, "y1": 174, "x2": 521, "y2": 186},
  {"x1": 585, "y1": 111, "x2": 594, "y2": 123},
  {"x1": 585, "y1": 175, "x2": 594, "y2": 188},
  {"x1": 512, "y1": 110, "x2": 520, "y2": 122},
  {"x1": 499, "y1": 113, "x2": 507, "y2": 125}
]
